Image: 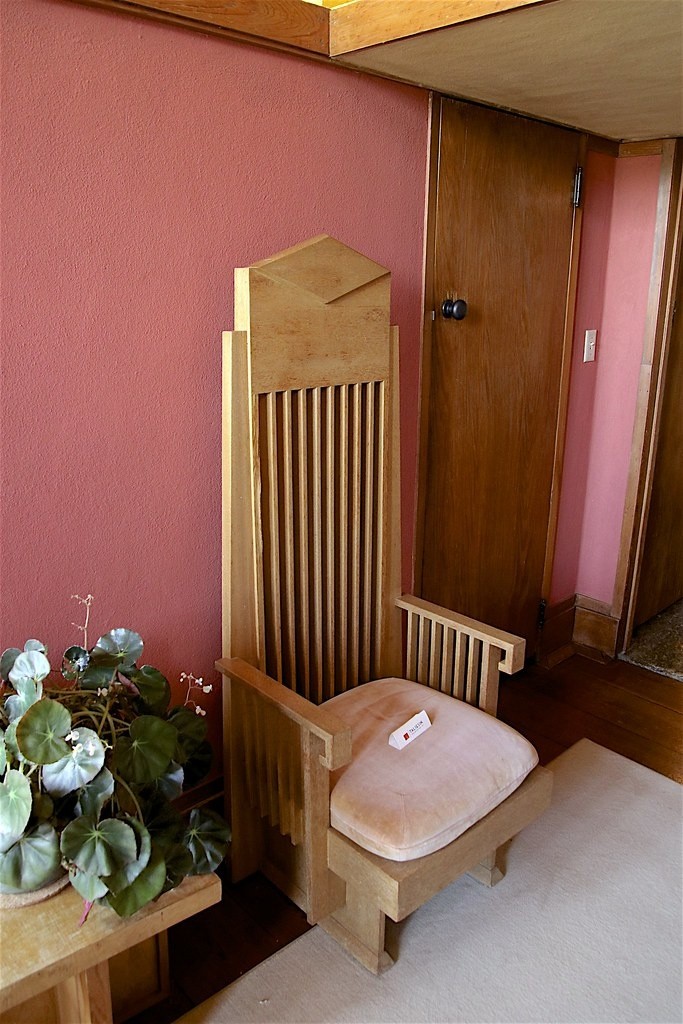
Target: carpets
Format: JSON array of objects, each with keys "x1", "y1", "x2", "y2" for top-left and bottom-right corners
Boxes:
[{"x1": 177, "y1": 738, "x2": 682, "y2": 1023}]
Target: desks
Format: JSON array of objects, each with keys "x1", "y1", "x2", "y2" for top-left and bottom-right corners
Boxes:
[{"x1": 1, "y1": 865, "x2": 223, "y2": 1024}]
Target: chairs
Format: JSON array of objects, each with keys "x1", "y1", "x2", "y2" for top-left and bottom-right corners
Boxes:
[{"x1": 213, "y1": 237, "x2": 554, "y2": 976}]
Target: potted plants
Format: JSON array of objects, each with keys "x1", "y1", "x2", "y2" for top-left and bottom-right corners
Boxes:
[{"x1": 0, "y1": 631, "x2": 230, "y2": 914}]
